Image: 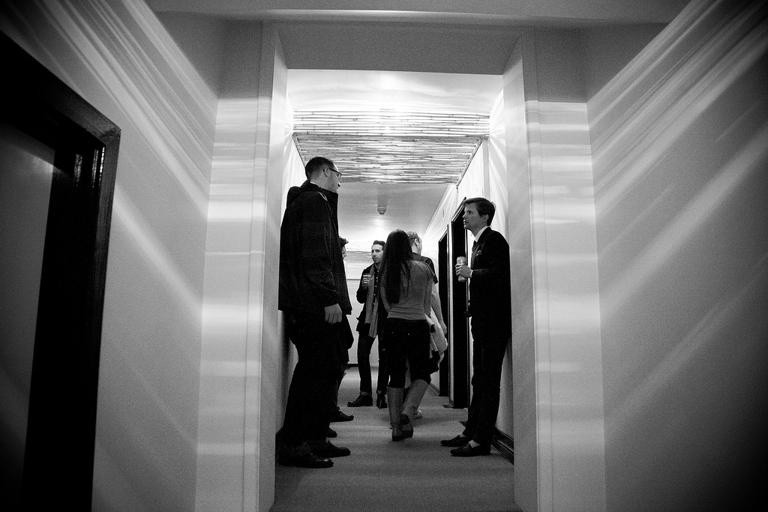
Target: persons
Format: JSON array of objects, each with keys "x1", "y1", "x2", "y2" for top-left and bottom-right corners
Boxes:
[
  {"x1": 440, "y1": 197, "x2": 510, "y2": 456},
  {"x1": 324, "y1": 230, "x2": 450, "y2": 443},
  {"x1": 275, "y1": 157, "x2": 352, "y2": 469}
]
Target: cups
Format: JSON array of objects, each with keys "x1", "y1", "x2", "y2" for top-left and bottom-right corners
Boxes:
[
  {"x1": 457, "y1": 256, "x2": 467, "y2": 282},
  {"x1": 363, "y1": 274, "x2": 371, "y2": 286}
]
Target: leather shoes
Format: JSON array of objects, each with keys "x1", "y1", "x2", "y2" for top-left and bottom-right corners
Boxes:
[
  {"x1": 325, "y1": 427, "x2": 337, "y2": 438},
  {"x1": 303, "y1": 451, "x2": 333, "y2": 468},
  {"x1": 322, "y1": 440, "x2": 351, "y2": 459},
  {"x1": 334, "y1": 407, "x2": 354, "y2": 422},
  {"x1": 441, "y1": 434, "x2": 467, "y2": 447},
  {"x1": 451, "y1": 440, "x2": 492, "y2": 457},
  {"x1": 375, "y1": 390, "x2": 387, "y2": 408},
  {"x1": 348, "y1": 391, "x2": 372, "y2": 406}
]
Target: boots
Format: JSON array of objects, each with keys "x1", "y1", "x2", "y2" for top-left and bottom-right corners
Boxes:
[
  {"x1": 401, "y1": 379, "x2": 429, "y2": 419},
  {"x1": 388, "y1": 386, "x2": 413, "y2": 442}
]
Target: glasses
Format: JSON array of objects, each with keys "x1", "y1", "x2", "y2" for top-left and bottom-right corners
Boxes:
[{"x1": 329, "y1": 166, "x2": 342, "y2": 178}]
